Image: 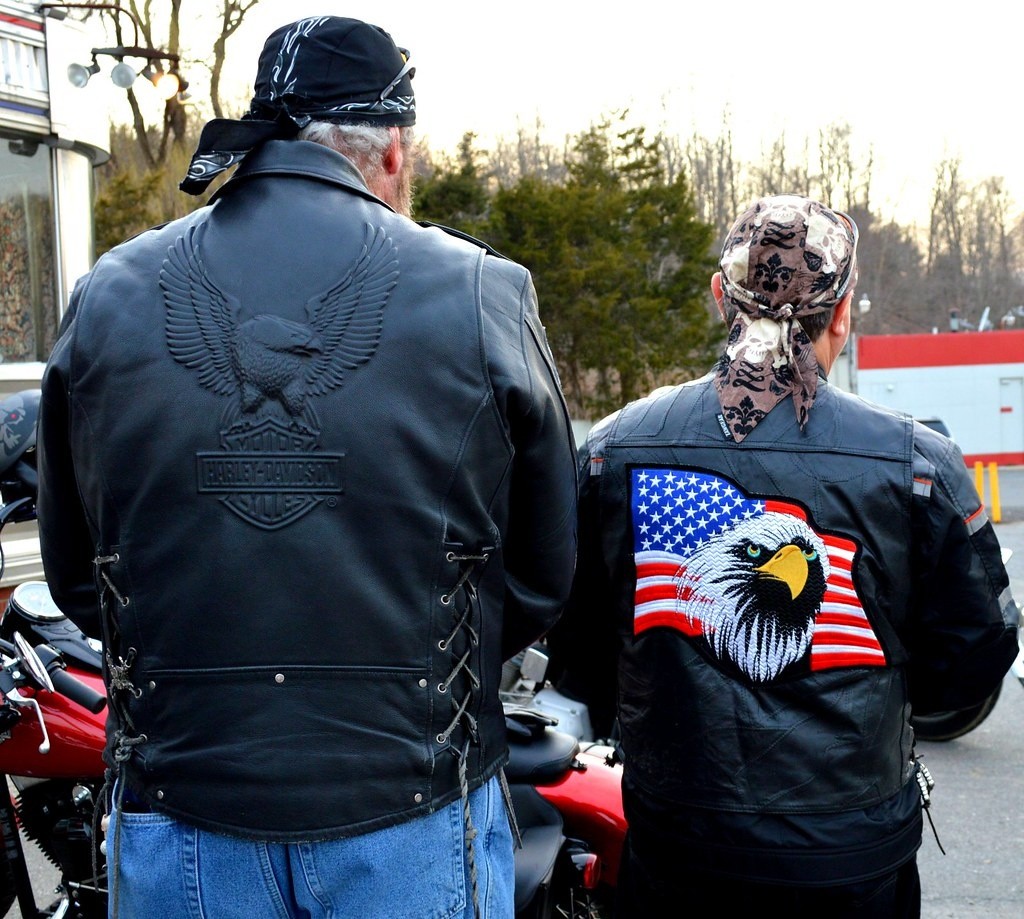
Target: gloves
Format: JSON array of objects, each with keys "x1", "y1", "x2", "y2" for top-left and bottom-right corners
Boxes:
[{"x1": 500, "y1": 702, "x2": 559, "y2": 737}]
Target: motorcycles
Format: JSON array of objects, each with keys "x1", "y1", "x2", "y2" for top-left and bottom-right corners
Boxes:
[{"x1": 0, "y1": 498, "x2": 623, "y2": 919}]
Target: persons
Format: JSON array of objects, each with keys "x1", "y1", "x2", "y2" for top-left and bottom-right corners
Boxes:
[
  {"x1": 550, "y1": 196, "x2": 1019, "y2": 919},
  {"x1": 39, "y1": 15, "x2": 586, "y2": 919}
]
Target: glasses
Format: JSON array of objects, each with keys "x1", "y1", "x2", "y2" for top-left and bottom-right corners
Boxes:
[
  {"x1": 380, "y1": 47, "x2": 416, "y2": 101},
  {"x1": 831, "y1": 209, "x2": 859, "y2": 300}
]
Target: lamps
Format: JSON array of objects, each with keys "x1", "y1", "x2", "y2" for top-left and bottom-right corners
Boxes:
[{"x1": 37, "y1": 3, "x2": 189, "y2": 99}]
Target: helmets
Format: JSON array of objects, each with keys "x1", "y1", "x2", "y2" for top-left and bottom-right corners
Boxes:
[{"x1": 0, "y1": 388, "x2": 44, "y2": 523}]
